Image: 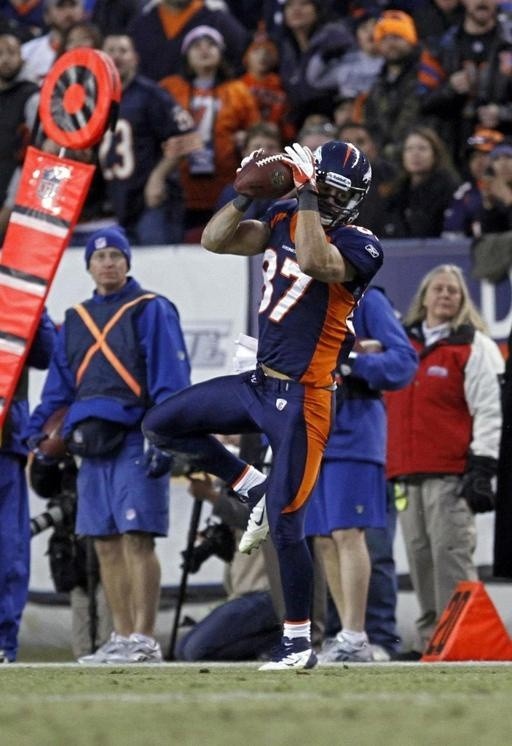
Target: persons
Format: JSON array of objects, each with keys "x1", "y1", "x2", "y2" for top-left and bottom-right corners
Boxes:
[
  {"x1": 14, "y1": 227, "x2": 192, "y2": 663},
  {"x1": 0, "y1": 309, "x2": 57, "y2": 662},
  {"x1": 172, "y1": 263, "x2": 505, "y2": 663},
  {"x1": 140, "y1": 140, "x2": 385, "y2": 669},
  {"x1": 0, "y1": 0, "x2": 512, "y2": 243}
]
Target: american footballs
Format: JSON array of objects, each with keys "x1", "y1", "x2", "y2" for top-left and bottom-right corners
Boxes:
[{"x1": 233, "y1": 153, "x2": 295, "y2": 201}]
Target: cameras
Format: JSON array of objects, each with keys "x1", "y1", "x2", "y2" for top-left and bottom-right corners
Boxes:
[{"x1": 181, "y1": 517, "x2": 237, "y2": 572}]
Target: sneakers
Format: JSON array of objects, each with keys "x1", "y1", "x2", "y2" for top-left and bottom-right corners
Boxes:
[
  {"x1": 228, "y1": 478, "x2": 269, "y2": 555},
  {"x1": 311, "y1": 638, "x2": 373, "y2": 662},
  {"x1": 259, "y1": 637, "x2": 317, "y2": 671},
  {"x1": 79, "y1": 639, "x2": 162, "y2": 664}
]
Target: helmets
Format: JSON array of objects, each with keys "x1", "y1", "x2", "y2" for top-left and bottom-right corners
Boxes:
[{"x1": 296, "y1": 140, "x2": 373, "y2": 227}]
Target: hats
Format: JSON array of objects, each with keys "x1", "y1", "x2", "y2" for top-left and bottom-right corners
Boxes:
[
  {"x1": 182, "y1": 25, "x2": 224, "y2": 52},
  {"x1": 373, "y1": 11, "x2": 417, "y2": 45},
  {"x1": 85, "y1": 228, "x2": 130, "y2": 269}
]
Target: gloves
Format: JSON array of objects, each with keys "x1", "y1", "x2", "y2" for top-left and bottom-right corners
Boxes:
[
  {"x1": 282, "y1": 143, "x2": 320, "y2": 194},
  {"x1": 236, "y1": 148, "x2": 264, "y2": 176},
  {"x1": 28, "y1": 434, "x2": 55, "y2": 466},
  {"x1": 144, "y1": 439, "x2": 174, "y2": 477},
  {"x1": 455, "y1": 469, "x2": 495, "y2": 514}
]
